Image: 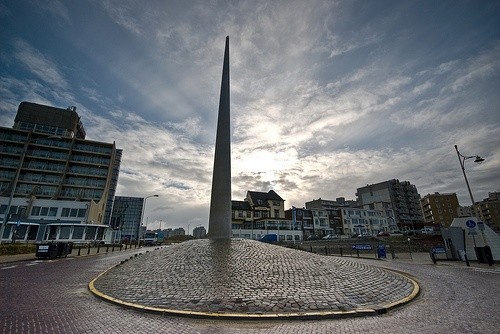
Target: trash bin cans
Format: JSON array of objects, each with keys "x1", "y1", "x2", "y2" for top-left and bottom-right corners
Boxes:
[
  {"x1": 34, "y1": 243, "x2": 61, "y2": 260},
  {"x1": 474, "y1": 247, "x2": 489, "y2": 264},
  {"x1": 57, "y1": 242, "x2": 74, "y2": 258}
]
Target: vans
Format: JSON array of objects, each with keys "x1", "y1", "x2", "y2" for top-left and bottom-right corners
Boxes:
[
  {"x1": 257, "y1": 234, "x2": 277, "y2": 242},
  {"x1": 144, "y1": 231, "x2": 162, "y2": 246}
]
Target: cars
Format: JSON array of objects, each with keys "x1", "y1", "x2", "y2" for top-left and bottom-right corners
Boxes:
[
  {"x1": 377, "y1": 231, "x2": 391, "y2": 237},
  {"x1": 357, "y1": 233, "x2": 372, "y2": 238},
  {"x1": 307, "y1": 234, "x2": 320, "y2": 240},
  {"x1": 321, "y1": 234, "x2": 338, "y2": 239}
]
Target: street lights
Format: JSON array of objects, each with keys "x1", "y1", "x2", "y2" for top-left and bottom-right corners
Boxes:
[
  {"x1": 0, "y1": 126, "x2": 61, "y2": 245},
  {"x1": 137, "y1": 194, "x2": 159, "y2": 248},
  {"x1": 454, "y1": 144, "x2": 494, "y2": 266}
]
